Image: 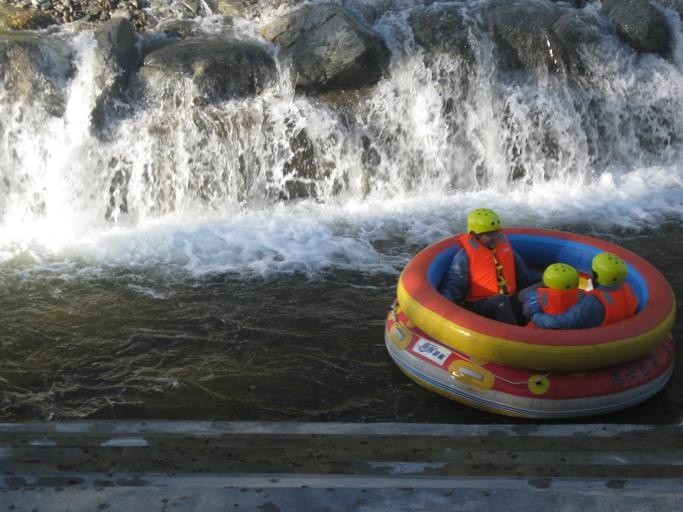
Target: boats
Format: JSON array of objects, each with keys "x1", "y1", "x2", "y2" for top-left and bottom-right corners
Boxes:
[{"x1": 383, "y1": 226, "x2": 678, "y2": 420}]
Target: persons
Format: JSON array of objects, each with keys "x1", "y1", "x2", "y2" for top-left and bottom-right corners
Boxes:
[
  {"x1": 523, "y1": 262, "x2": 588, "y2": 330},
  {"x1": 521, "y1": 251, "x2": 638, "y2": 330},
  {"x1": 437, "y1": 209, "x2": 541, "y2": 325}
]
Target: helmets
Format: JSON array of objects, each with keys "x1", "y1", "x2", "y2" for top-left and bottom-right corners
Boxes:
[
  {"x1": 467, "y1": 207, "x2": 502, "y2": 234},
  {"x1": 591, "y1": 251, "x2": 625, "y2": 288},
  {"x1": 543, "y1": 263, "x2": 578, "y2": 290}
]
